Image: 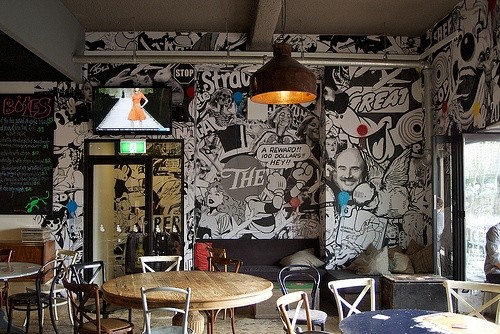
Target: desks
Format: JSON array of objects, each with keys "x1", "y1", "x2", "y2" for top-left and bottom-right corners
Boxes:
[
  {"x1": 101, "y1": 271, "x2": 274, "y2": 334},
  {"x1": 0, "y1": 262, "x2": 45, "y2": 321},
  {"x1": 339, "y1": 309, "x2": 500, "y2": 334}
]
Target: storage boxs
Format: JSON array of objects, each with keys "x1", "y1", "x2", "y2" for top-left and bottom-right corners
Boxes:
[{"x1": 248, "y1": 281, "x2": 320, "y2": 318}]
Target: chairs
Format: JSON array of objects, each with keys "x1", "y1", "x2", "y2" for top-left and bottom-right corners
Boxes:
[
  {"x1": 442, "y1": 280, "x2": 500, "y2": 325},
  {"x1": 137, "y1": 247, "x2": 375, "y2": 334},
  {"x1": 0, "y1": 250, "x2": 135, "y2": 334}
]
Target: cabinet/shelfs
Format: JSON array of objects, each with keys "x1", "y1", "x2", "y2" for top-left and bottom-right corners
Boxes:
[{"x1": 0, "y1": 239, "x2": 55, "y2": 285}]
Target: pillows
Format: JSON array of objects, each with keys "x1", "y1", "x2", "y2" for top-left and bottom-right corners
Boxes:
[
  {"x1": 194, "y1": 243, "x2": 213, "y2": 271},
  {"x1": 345, "y1": 239, "x2": 434, "y2": 275},
  {"x1": 280, "y1": 249, "x2": 326, "y2": 271}
]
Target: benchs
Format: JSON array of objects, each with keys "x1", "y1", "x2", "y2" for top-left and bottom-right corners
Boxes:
[
  {"x1": 196, "y1": 238, "x2": 327, "y2": 280},
  {"x1": 382, "y1": 273, "x2": 455, "y2": 312},
  {"x1": 325, "y1": 269, "x2": 382, "y2": 316}
]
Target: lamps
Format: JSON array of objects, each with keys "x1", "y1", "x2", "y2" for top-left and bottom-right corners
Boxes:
[
  {"x1": 219, "y1": 18, "x2": 234, "y2": 72},
  {"x1": 127, "y1": 17, "x2": 142, "y2": 74},
  {"x1": 250, "y1": 0, "x2": 317, "y2": 104}
]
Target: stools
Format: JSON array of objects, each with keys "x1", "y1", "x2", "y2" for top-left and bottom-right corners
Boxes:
[{"x1": 481, "y1": 281, "x2": 496, "y2": 317}]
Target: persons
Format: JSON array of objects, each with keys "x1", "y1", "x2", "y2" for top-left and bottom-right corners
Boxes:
[
  {"x1": 121, "y1": 88, "x2": 149, "y2": 128},
  {"x1": 484, "y1": 223, "x2": 500, "y2": 285}
]
